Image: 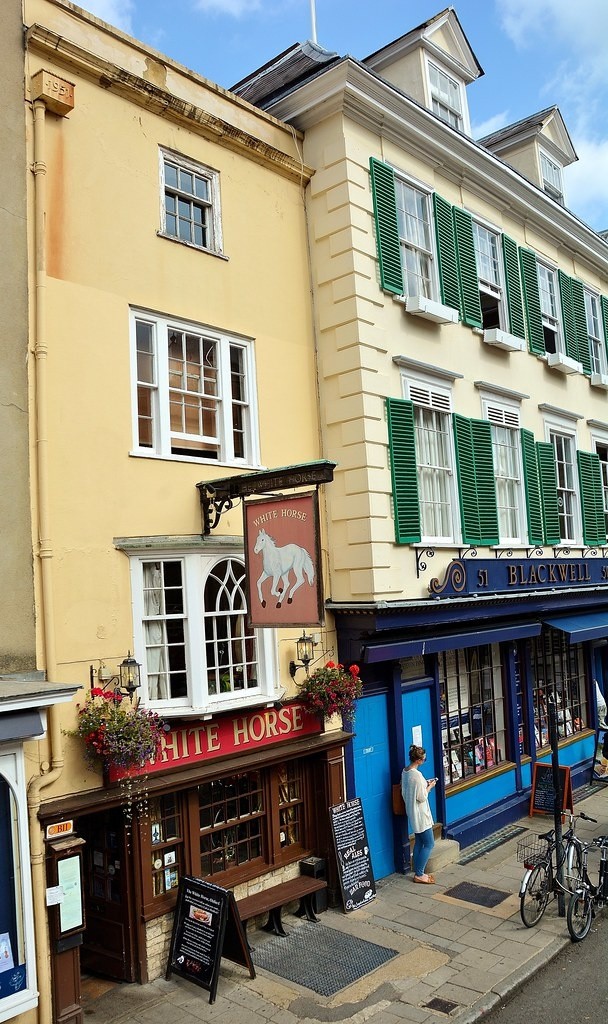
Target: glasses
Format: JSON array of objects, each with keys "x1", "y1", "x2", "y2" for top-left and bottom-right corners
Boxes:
[{"x1": 422, "y1": 758, "x2": 426, "y2": 761}]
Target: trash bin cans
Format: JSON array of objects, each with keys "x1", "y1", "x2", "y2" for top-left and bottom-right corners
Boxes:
[{"x1": 299, "y1": 856, "x2": 328, "y2": 914}]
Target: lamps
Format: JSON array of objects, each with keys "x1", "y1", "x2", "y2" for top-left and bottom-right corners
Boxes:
[
  {"x1": 89, "y1": 649, "x2": 140, "y2": 705},
  {"x1": 289, "y1": 629, "x2": 313, "y2": 678}
]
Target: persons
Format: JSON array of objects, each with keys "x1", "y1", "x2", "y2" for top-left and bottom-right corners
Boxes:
[{"x1": 401, "y1": 745, "x2": 436, "y2": 884}]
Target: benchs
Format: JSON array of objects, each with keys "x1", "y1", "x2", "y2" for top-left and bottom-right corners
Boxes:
[{"x1": 236, "y1": 874, "x2": 327, "y2": 954}]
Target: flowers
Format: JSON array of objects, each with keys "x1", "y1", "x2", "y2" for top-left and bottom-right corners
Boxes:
[
  {"x1": 298, "y1": 660, "x2": 364, "y2": 722},
  {"x1": 66, "y1": 688, "x2": 165, "y2": 845}
]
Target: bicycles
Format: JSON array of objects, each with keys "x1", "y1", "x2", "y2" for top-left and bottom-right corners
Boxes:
[
  {"x1": 519, "y1": 812, "x2": 598, "y2": 929},
  {"x1": 566, "y1": 836, "x2": 608, "y2": 942}
]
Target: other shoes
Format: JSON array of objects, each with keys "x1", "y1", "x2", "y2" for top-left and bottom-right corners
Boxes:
[{"x1": 413, "y1": 876, "x2": 435, "y2": 884}]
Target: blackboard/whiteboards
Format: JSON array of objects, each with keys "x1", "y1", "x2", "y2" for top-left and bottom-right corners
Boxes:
[
  {"x1": 328, "y1": 797, "x2": 378, "y2": 914},
  {"x1": 529, "y1": 762, "x2": 569, "y2": 815}
]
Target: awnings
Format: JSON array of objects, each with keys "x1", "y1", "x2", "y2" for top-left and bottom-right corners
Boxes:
[{"x1": 544, "y1": 615, "x2": 608, "y2": 645}]
